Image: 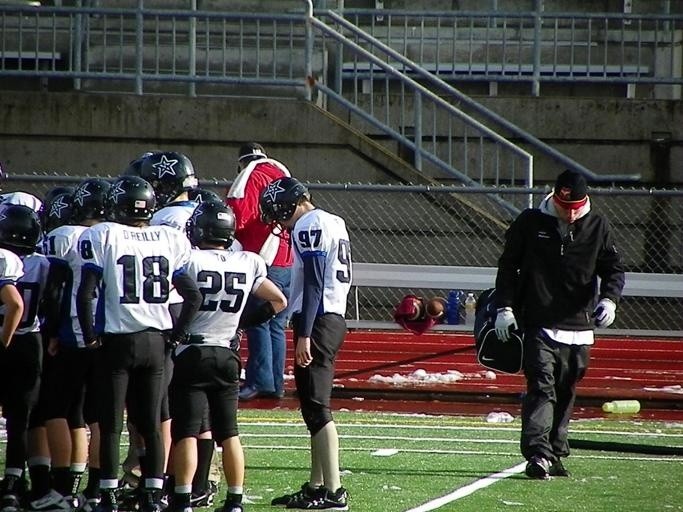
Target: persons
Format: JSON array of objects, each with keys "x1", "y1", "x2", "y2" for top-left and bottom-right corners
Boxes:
[
  {"x1": 25, "y1": 188, "x2": 88, "y2": 512},
  {"x1": 77, "y1": 176, "x2": 203, "y2": 512},
  {"x1": 142, "y1": 151, "x2": 234, "y2": 508},
  {"x1": 32, "y1": 180, "x2": 111, "y2": 512},
  {"x1": 259, "y1": 177, "x2": 353, "y2": 511},
  {"x1": 165, "y1": 199, "x2": 288, "y2": 512},
  {"x1": 0, "y1": 246, "x2": 24, "y2": 354},
  {"x1": 224, "y1": 143, "x2": 295, "y2": 400},
  {"x1": 0, "y1": 205, "x2": 49, "y2": 510},
  {"x1": 497, "y1": 170, "x2": 626, "y2": 478}
]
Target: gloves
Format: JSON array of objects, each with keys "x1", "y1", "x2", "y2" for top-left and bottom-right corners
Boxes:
[
  {"x1": 182, "y1": 333, "x2": 204, "y2": 346},
  {"x1": 164, "y1": 334, "x2": 181, "y2": 352},
  {"x1": 592, "y1": 299, "x2": 618, "y2": 329},
  {"x1": 229, "y1": 299, "x2": 279, "y2": 353},
  {"x1": 496, "y1": 306, "x2": 520, "y2": 342}
]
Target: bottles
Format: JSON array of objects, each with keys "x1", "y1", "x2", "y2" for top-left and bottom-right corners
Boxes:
[
  {"x1": 602, "y1": 400, "x2": 641, "y2": 414},
  {"x1": 465, "y1": 293, "x2": 476, "y2": 327}
]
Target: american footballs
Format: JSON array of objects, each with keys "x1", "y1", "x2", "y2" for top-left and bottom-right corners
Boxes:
[
  {"x1": 424, "y1": 296, "x2": 448, "y2": 324},
  {"x1": 408, "y1": 299, "x2": 424, "y2": 321}
]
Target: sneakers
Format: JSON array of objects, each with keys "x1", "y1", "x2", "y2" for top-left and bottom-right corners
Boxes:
[
  {"x1": 239, "y1": 383, "x2": 276, "y2": 402},
  {"x1": 296, "y1": 486, "x2": 350, "y2": 511},
  {"x1": 188, "y1": 490, "x2": 213, "y2": 508},
  {"x1": 270, "y1": 482, "x2": 317, "y2": 509},
  {"x1": 213, "y1": 501, "x2": 244, "y2": 512},
  {"x1": 22, "y1": 490, "x2": 75, "y2": 512},
  {"x1": 163, "y1": 505, "x2": 194, "y2": 512},
  {"x1": 0, "y1": 489, "x2": 20, "y2": 512},
  {"x1": 97, "y1": 491, "x2": 119, "y2": 512},
  {"x1": 526, "y1": 457, "x2": 550, "y2": 478},
  {"x1": 123, "y1": 491, "x2": 162, "y2": 512},
  {"x1": 116, "y1": 473, "x2": 139, "y2": 506},
  {"x1": 551, "y1": 462, "x2": 573, "y2": 477},
  {"x1": 73, "y1": 492, "x2": 101, "y2": 512}
]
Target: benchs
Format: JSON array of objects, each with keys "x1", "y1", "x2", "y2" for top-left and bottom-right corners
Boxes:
[
  {"x1": 342, "y1": 63, "x2": 653, "y2": 98},
  {"x1": 0, "y1": 51, "x2": 66, "y2": 88}
]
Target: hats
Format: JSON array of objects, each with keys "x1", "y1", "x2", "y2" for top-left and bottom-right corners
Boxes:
[
  {"x1": 236, "y1": 143, "x2": 266, "y2": 174},
  {"x1": 551, "y1": 168, "x2": 589, "y2": 210}
]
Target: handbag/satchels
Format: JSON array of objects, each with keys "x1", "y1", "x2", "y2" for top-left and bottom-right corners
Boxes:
[{"x1": 472, "y1": 207, "x2": 542, "y2": 377}]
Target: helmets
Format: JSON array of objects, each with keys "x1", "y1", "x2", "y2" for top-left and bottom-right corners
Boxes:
[
  {"x1": 70, "y1": 177, "x2": 113, "y2": 221},
  {"x1": 141, "y1": 149, "x2": 198, "y2": 206},
  {"x1": 104, "y1": 175, "x2": 157, "y2": 222},
  {"x1": 259, "y1": 178, "x2": 307, "y2": 243},
  {"x1": 1, "y1": 204, "x2": 40, "y2": 255},
  {"x1": 44, "y1": 185, "x2": 75, "y2": 231},
  {"x1": 184, "y1": 198, "x2": 237, "y2": 249}
]
